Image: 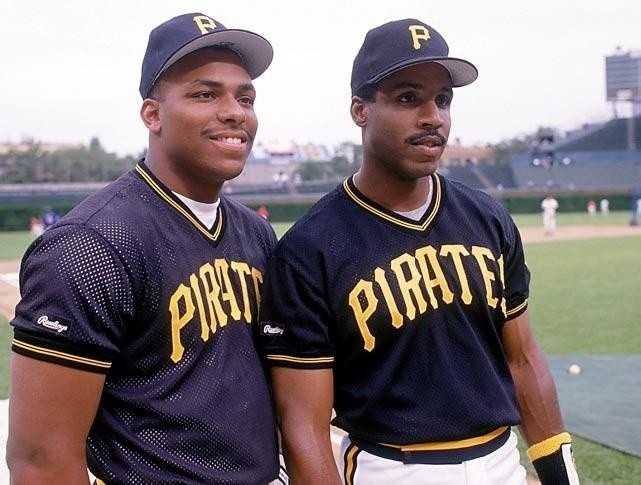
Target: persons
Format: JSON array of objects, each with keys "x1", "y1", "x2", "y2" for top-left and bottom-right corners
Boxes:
[
  {"x1": 6, "y1": 12, "x2": 276, "y2": 484},
  {"x1": 42, "y1": 205, "x2": 60, "y2": 229},
  {"x1": 30, "y1": 216, "x2": 45, "y2": 238},
  {"x1": 541, "y1": 194, "x2": 559, "y2": 234},
  {"x1": 257, "y1": 19, "x2": 582, "y2": 484},
  {"x1": 257, "y1": 203, "x2": 269, "y2": 221},
  {"x1": 586, "y1": 198, "x2": 610, "y2": 217}
]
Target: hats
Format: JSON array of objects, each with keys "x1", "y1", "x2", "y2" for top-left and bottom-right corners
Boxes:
[
  {"x1": 350, "y1": 18, "x2": 478, "y2": 98},
  {"x1": 139, "y1": 13, "x2": 274, "y2": 98}
]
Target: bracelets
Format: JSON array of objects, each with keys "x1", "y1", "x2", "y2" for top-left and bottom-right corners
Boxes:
[{"x1": 525, "y1": 431, "x2": 582, "y2": 485}]
